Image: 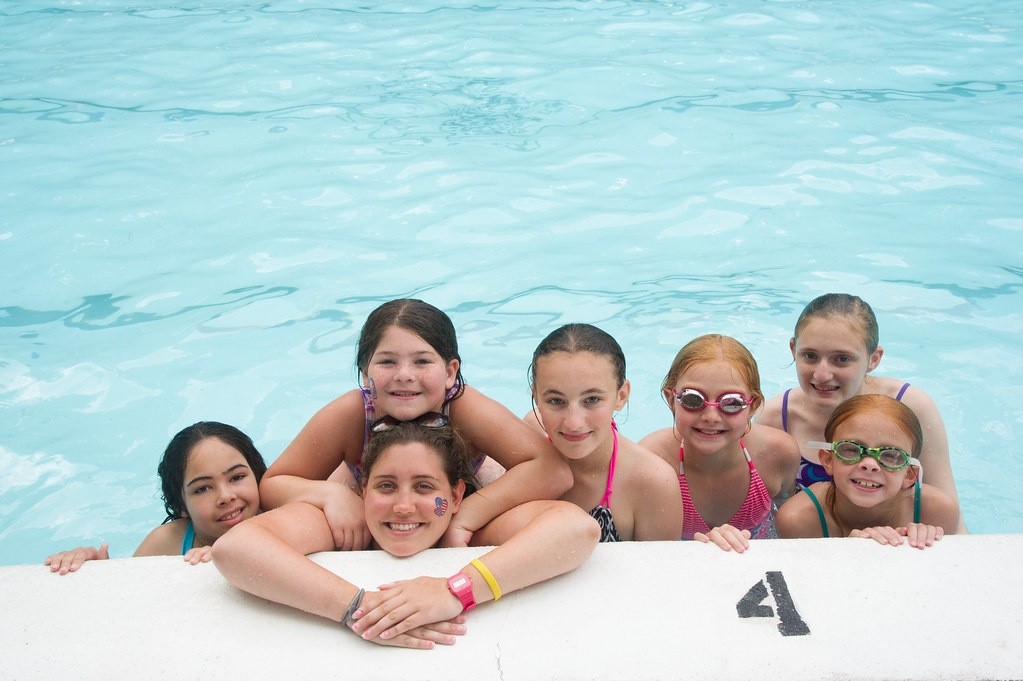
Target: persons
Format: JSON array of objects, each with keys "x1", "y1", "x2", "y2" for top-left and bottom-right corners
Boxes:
[
  {"x1": 692, "y1": 398, "x2": 699, "y2": 408},
  {"x1": 259, "y1": 299, "x2": 574, "y2": 552},
  {"x1": 211, "y1": 411, "x2": 600, "y2": 650},
  {"x1": 523, "y1": 322, "x2": 684, "y2": 542},
  {"x1": 638, "y1": 333, "x2": 802, "y2": 554},
  {"x1": 777, "y1": 393, "x2": 959, "y2": 548},
  {"x1": 45, "y1": 419, "x2": 267, "y2": 575},
  {"x1": 752, "y1": 293, "x2": 959, "y2": 503}
]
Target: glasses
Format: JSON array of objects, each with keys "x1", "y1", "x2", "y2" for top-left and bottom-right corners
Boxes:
[
  {"x1": 671, "y1": 386, "x2": 754, "y2": 415},
  {"x1": 803, "y1": 439, "x2": 921, "y2": 471}
]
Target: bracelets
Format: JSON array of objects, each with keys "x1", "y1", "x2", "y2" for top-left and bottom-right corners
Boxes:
[
  {"x1": 341, "y1": 588, "x2": 365, "y2": 625},
  {"x1": 470, "y1": 559, "x2": 502, "y2": 601}
]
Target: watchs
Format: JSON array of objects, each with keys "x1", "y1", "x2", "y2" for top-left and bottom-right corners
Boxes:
[{"x1": 447, "y1": 572, "x2": 476, "y2": 612}]
